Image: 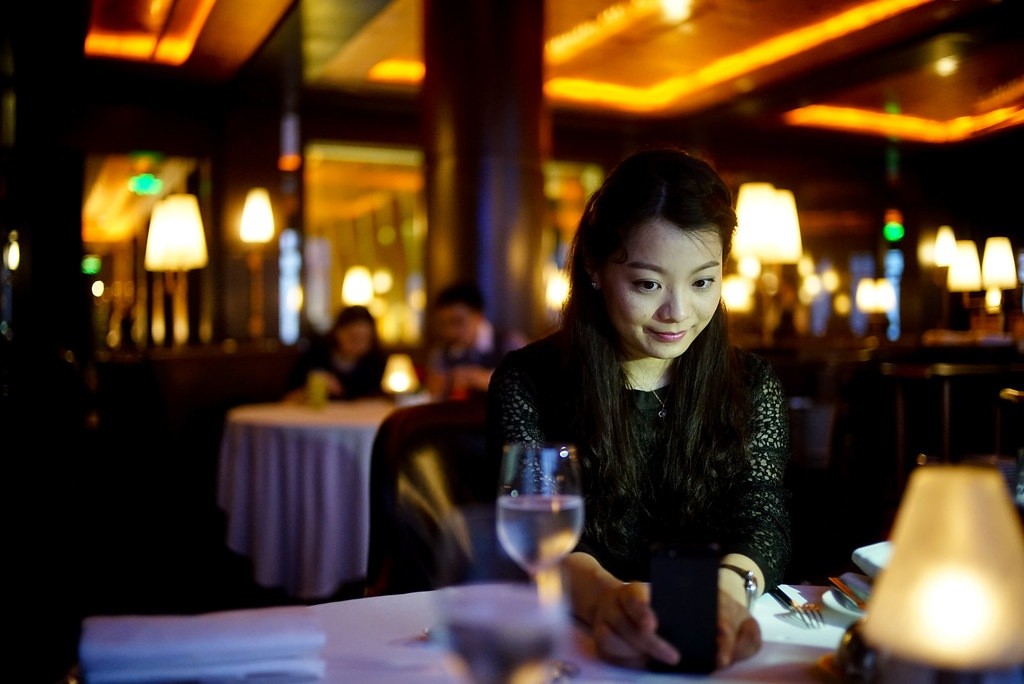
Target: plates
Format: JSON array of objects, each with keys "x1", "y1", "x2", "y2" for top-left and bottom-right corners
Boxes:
[{"x1": 821, "y1": 586, "x2": 868, "y2": 619}]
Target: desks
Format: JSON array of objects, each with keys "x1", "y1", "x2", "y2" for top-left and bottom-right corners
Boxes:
[
  {"x1": 879, "y1": 363, "x2": 1005, "y2": 464},
  {"x1": 306, "y1": 585, "x2": 855, "y2": 684},
  {"x1": 215, "y1": 399, "x2": 394, "y2": 599}
]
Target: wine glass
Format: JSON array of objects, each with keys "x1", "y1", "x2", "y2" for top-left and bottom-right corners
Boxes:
[{"x1": 495, "y1": 442, "x2": 585, "y2": 684}]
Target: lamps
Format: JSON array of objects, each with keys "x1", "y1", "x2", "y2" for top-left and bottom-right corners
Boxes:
[{"x1": 858, "y1": 462, "x2": 1024, "y2": 684}]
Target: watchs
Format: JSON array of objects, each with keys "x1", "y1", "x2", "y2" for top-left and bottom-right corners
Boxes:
[{"x1": 720, "y1": 563, "x2": 758, "y2": 609}]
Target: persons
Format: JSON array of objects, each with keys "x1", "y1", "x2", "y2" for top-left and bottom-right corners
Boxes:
[
  {"x1": 283, "y1": 306, "x2": 389, "y2": 405},
  {"x1": 485, "y1": 147, "x2": 789, "y2": 669},
  {"x1": 422, "y1": 282, "x2": 529, "y2": 392}
]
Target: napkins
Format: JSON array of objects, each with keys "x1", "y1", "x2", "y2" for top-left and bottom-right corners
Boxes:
[{"x1": 80, "y1": 607, "x2": 329, "y2": 683}]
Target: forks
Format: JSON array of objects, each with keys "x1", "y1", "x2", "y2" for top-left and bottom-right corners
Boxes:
[{"x1": 770, "y1": 586, "x2": 826, "y2": 629}]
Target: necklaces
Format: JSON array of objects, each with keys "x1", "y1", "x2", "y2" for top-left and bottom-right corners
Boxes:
[{"x1": 620, "y1": 358, "x2": 683, "y2": 421}]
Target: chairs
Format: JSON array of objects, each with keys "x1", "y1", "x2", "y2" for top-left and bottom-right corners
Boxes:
[{"x1": 369, "y1": 395, "x2": 486, "y2": 596}]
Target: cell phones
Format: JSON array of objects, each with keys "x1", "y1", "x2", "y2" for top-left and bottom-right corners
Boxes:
[{"x1": 650, "y1": 541, "x2": 720, "y2": 675}]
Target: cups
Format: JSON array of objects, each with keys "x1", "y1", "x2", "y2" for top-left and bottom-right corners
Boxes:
[
  {"x1": 306, "y1": 369, "x2": 330, "y2": 411},
  {"x1": 426, "y1": 582, "x2": 563, "y2": 684},
  {"x1": 647, "y1": 541, "x2": 720, "y2": 675}
]
